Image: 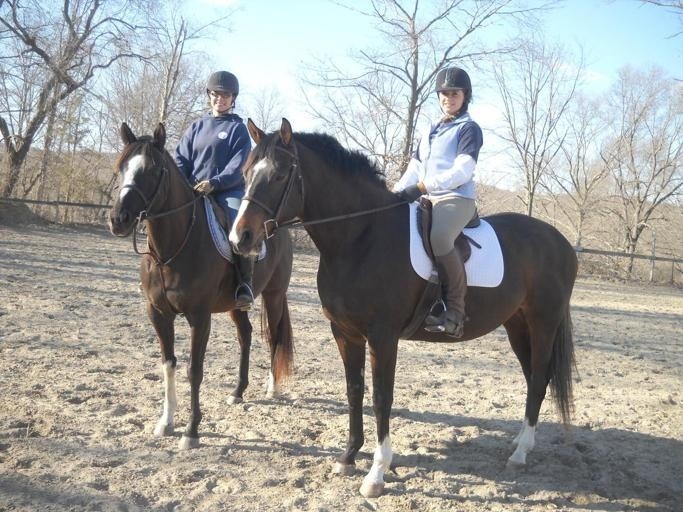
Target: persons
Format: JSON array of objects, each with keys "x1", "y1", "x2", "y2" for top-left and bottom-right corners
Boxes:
[
  {"x1": 390, "y1": 68, "x2": 483, "y2": 337},
  {"x1": 171, "y1": 70, "x2": 254, "y2": 314}
]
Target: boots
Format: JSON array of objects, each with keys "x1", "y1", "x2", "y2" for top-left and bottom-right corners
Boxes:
[
  {"x1": 425, "y1": 248, "x2": 468, "y2": 339},
  {"x1": 234, "y1": 253, "x2": 255, "y2": 310}
]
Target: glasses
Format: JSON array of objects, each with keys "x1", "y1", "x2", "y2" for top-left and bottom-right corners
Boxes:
[{"x1": 210, "y1": 92, "x2": 231, "y2": 99}]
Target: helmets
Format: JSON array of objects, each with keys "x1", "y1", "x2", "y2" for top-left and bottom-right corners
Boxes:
[
  {"x1": 436, "y1": 68, "x2": 471, "y2": 91},
  {"x1": 207, "y1": 71, "x2": 238, "y2": 96}
]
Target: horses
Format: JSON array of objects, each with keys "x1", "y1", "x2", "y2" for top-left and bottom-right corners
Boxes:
[
  {"x1": 228, "y1": 117, "x2": 581, "y2": 499},
  {"x1": 108, "y1": 121, "x2": 298, "y2": 451}
]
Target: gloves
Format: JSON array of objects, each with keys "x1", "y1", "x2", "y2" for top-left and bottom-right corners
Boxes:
[{"x1": 399, "y1": 185, "x2": 423, "y2": 204}]
